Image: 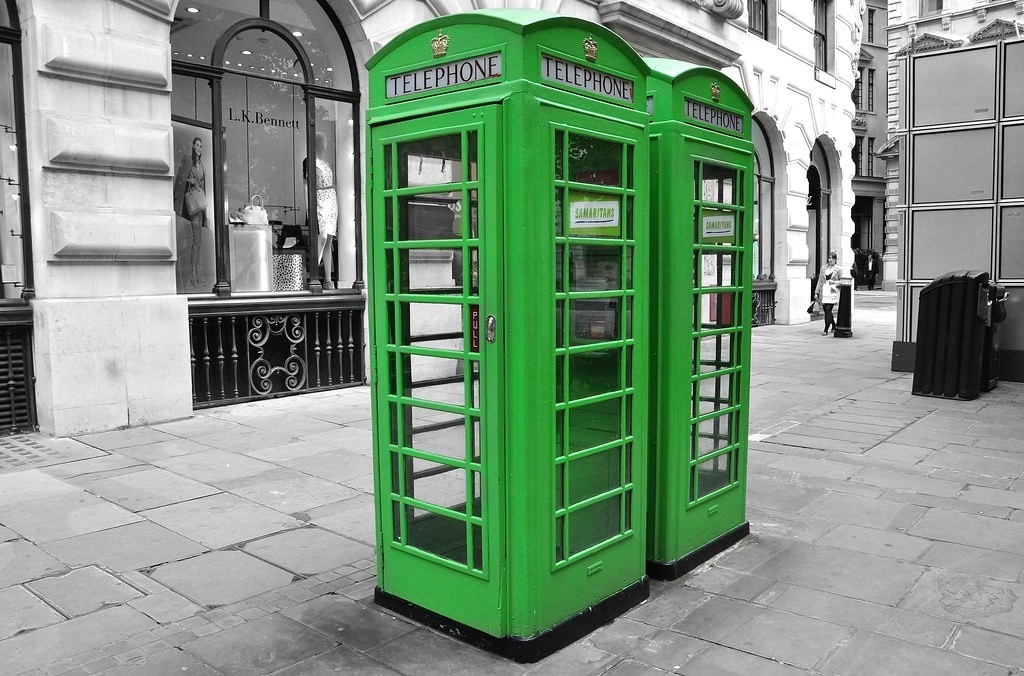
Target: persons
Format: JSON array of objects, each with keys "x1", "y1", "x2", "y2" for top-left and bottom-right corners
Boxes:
[
  {"x1": 173, "y1": 136, "x2": 207, "y2": 287},
  {"x1": 864, "y1": 254, "x2": 879, "y2": 290},
  {"x1": 303, "y1": 132, "x2": 338, "y2": 290},
  {"x1": 814, "y1": 252, "x2": 842, "y2": 335}
]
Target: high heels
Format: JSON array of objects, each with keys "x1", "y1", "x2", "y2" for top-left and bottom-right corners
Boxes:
[{"x1": 190, "y1": 274, "x2": 206, "y2": 287}]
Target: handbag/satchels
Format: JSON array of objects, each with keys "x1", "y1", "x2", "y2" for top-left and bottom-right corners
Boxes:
[
  {"x1": 821, "y1": 280, "x2": 838, "y2": 304},
  {"x1": 240, "y1": 194, "x2": 268, "y2": 227},
  {"x1": 185, "y1": 181, "x2": 207, "y2": 217},
  {"x1": 281, "y1": 223, "x2": 303, "y2": 241},
  {"x1": 808, "y1": 298, "x2": 819, "y2": 313}
]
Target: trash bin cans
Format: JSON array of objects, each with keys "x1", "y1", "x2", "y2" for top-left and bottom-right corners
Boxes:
[
  {"x1": 910, "y1": 272, "x2": 990, "y2": 402},
  {"x1": 981, "y1": 284, "x2": 1006, "y2": 393}
]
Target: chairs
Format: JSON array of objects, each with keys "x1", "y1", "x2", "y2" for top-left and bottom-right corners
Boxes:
[{"x1": 273, "y1": 249, "x2": 306, "y2": 292}]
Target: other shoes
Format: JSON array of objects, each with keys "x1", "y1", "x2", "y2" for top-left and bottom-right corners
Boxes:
[
  {"x1": 822, "y1": 331, "x2": 828, "y2": 336},
  {"x1": 229, "y1": 211, "x2": 245, "y2": 224},
  {"x1": 832, "y1": 325, "x2": 836, "y2": 332}
]
